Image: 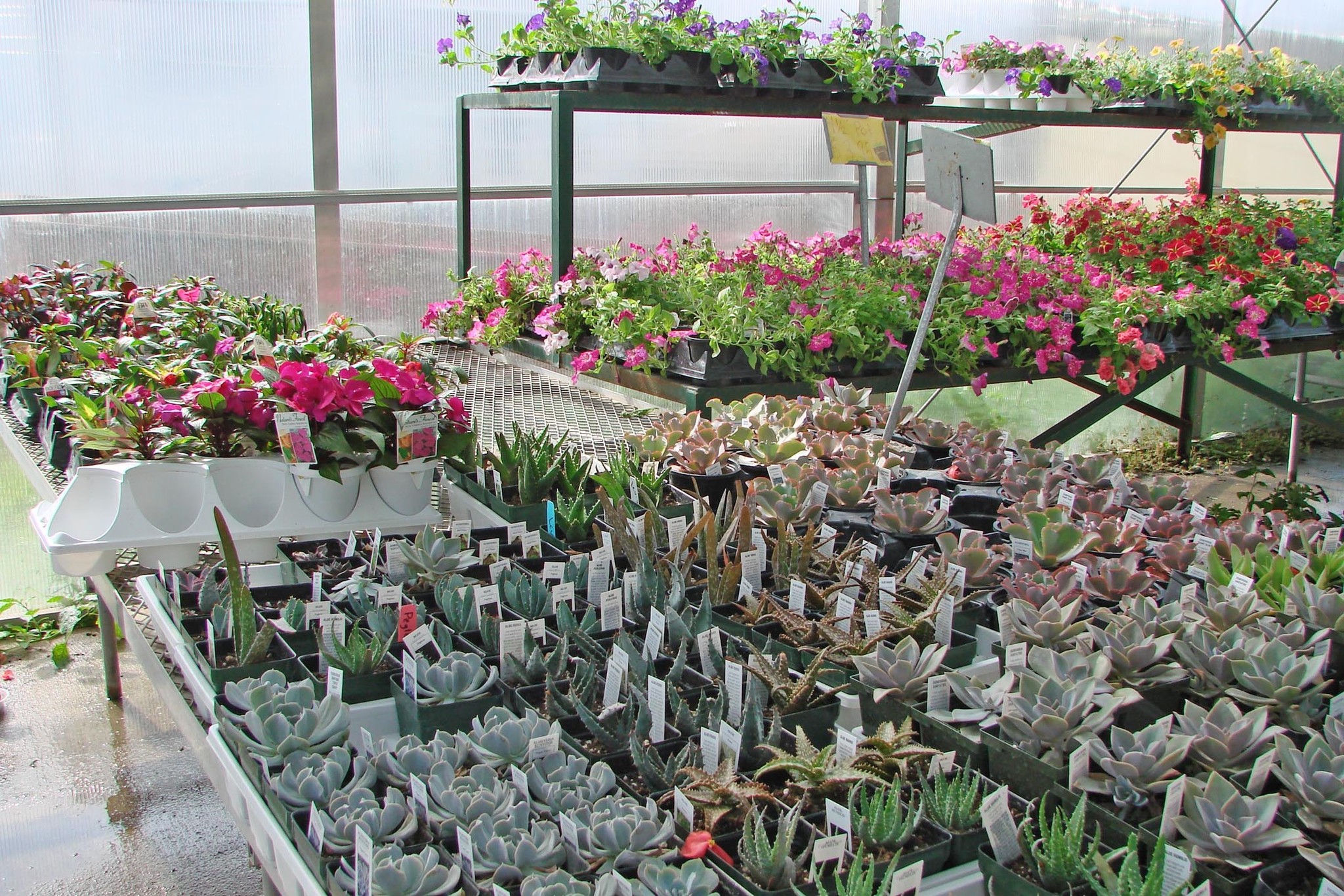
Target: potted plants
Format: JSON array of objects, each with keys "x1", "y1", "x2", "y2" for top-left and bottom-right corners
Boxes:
[{"x1": 136, "y1": 378, "x2": 1344, "y2": 896}]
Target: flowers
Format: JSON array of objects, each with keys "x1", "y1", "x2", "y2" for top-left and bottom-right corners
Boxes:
[
  {"x1": 437, "y1": 0, "x2": 1344, "y2": 161},
  {"x1": 0, "y1": 177, "x2": 1344, "y2": 485}
]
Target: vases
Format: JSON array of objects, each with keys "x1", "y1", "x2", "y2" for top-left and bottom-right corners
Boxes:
[
  {"x1": 1045, "y1": 75, "x2": 1071, "y2": 94},
  {"x1": 289, "y1": 455, "x2": 366, "y2": 522},
  {"x1": 517, "y1": 57, "x2": 540, "y2": 91},
  {"x1": 563, "y1": 53, "x2": 587, "y2": 90},
  {"x1": 668, "y1": 50, "x2": 701, "y2": 93},
  {"x1": 685, "y1": 334, "x2": 733, "y2": 369},
  {"x1": 982, "y1": 69, "x2": 1009, "y2": 109},
  {"x1": 496, "y1": 56, "x2": 523, "y2": 92},
  {"x1": 539, "y1": 52, "x2": 562, "y2": 90},
  {"x1": 584, "y1": 46, "x2": 627, "y2": 91},
  {"x1": 364, "y1": 453, "x2": 438, "y2": 515},
  {"x1": 958, "y1": 70, "x2": 983, "y2": 106},
  {"x1": 907, "y1": 65, "x2": 938, "y2": 105}
]
